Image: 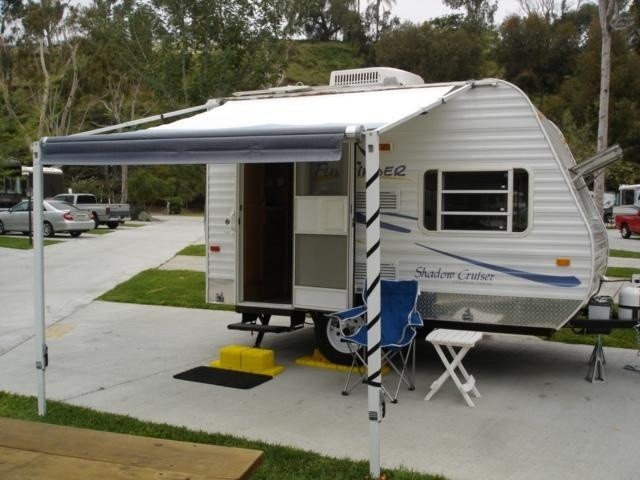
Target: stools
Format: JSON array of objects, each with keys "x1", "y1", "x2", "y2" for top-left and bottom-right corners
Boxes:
[{"x1": 421, "y1": 325, "x2": 484, "y2": 407}]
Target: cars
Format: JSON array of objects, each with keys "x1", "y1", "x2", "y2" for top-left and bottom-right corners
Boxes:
[{"x1": 0, "y1": 197, "x2": 97, "y2": 238}]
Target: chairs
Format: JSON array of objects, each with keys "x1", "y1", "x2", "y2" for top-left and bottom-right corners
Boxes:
[{"x1": 328, "y1": 275, "x2": 424, "y2": 403}]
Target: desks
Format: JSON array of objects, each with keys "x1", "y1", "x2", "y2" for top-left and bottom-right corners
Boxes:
[{"x1": 0, "y1": 415, "x2": 266, "y2": 480}]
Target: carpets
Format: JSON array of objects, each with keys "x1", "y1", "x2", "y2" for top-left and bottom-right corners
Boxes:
[{"x1": 173, "y1": 364, "x2": 271, "y2": 392}]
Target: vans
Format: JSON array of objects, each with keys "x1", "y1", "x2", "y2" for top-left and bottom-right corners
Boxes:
[{"x1": 611, "y1": 183, "x2": 640, "y2": 240}]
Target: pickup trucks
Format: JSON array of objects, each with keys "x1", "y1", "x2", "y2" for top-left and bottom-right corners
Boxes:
[{"x1": 50, "y1": 193, "x2": 131, "y2": 232}]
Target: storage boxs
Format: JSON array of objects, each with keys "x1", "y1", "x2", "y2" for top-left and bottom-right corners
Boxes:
[{"x1": 586, "y1": 297, "x2": 615, "y2": 319}]
